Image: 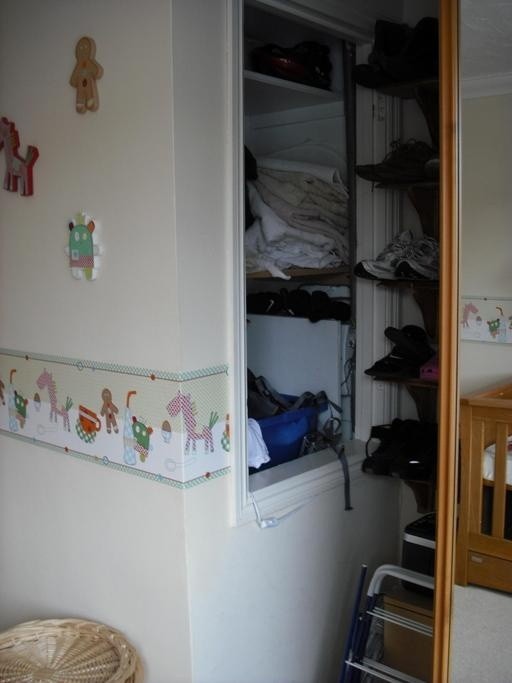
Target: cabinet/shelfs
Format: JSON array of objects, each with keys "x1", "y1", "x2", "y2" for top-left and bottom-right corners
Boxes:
[
  {"x1": 374, "y1": 78, "x2": 440, "y2": 483},
  {"x1": 227, "y1": 0, "x2": 380, "y2": 527}
]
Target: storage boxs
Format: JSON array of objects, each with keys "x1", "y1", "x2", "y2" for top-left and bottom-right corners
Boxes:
[{"x1": 247, "y1": 394, "x2": 329, "y2": 476}]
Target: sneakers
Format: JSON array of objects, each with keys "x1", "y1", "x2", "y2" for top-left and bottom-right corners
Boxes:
[
  {"x1": 357, "y1": 228, "x2": 416, "y2": 281},
  {"x1": 368, "y1": 138, "x2": 434, "y2": 182},
  {"x1": 355, "y1": 139, "x2": 413, "y2": 184},
  {"x1": 364, "y1": 326, "x2": 440, "y2": 481},
  {"x1": 393, "y1": 236, "x2": 439, "y2": 281}
]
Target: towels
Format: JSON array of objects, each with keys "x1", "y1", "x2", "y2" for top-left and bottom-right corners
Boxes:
[{"x1": 246, "y1": 418, "x2": 270, "y2": 469}]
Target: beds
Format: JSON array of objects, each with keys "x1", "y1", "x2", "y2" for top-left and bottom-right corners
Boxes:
[{"x1": 455, "y1": 381, "x2": 512, "y2": 594}]
